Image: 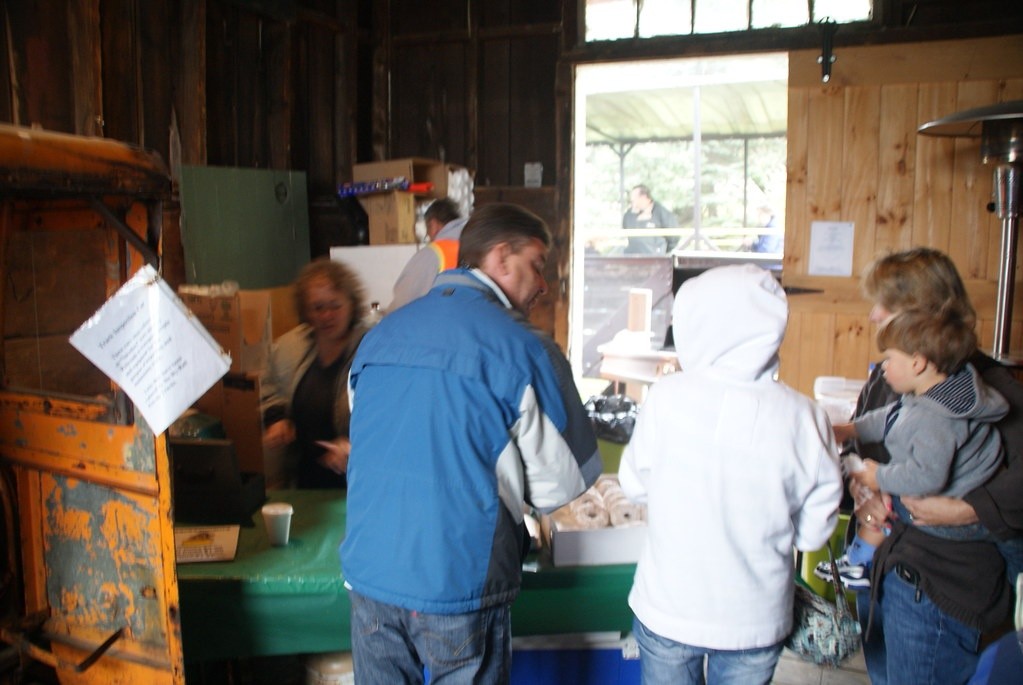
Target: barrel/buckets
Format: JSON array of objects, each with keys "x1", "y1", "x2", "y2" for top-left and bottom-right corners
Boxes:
[{"x1": 814, "y1": 376, "x2": 867, "y2": 425}]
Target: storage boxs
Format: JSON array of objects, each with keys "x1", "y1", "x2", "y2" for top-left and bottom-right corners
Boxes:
[
  {"x1": 175, "y1": 283, "x2": 306, "y2": 480},
  {"x1": 353, "y1": 158, "x2": 477, "y2": 246}
]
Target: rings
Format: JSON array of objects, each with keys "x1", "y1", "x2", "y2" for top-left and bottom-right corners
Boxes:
[{"x1": 864, "y1": 513, "x2": 871, "y2": 522}]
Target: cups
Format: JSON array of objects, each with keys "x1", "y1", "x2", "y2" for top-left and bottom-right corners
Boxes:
[
  {"x1": 262, "y1": 503, "x2": 292, "y2": 546},
  {"x1": 628, "y1": 285, "x2": 652, "y2": 333},
  {"x1": 523, "y1": 162, "x2": 543, "y2": 187}
]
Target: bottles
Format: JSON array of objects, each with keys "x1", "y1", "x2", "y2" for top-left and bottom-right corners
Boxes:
[{"x1": 839, "y1": 446, "x2": 880, "y2": 493}]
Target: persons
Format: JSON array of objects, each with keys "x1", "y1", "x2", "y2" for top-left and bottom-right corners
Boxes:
[
  {"x1": 338, "y1": 203, "x2": 603, "y2": 685},
  {"x1": 839, "y1": 247, "x2": 1023, "y2": 685},
  {"x1": 618, "y1": 263, "x2": 844, "y2": 684},
  {"x1": 620, "y1": 185, "x2": 680, "y2": 254},
  {"x1": 813, "y1": 301, "x2": 1010, "y2": 591},
  {"x1": 387, "y1": 197, "x2": 469, "y2": 309},
  {"x1": 258, "y1": 260, "x2": 371, "y2": 489}
]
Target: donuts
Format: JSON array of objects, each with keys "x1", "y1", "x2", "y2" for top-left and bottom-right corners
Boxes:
[{"x1": 566, "y1": 480, "x2": 646, "y2": 528}]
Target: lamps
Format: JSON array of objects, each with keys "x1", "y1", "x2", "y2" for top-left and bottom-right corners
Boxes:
[{"x1": 915, "y1": 101, "x2": 1023, "y2": 364}]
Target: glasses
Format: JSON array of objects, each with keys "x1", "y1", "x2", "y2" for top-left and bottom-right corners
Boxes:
[{"x1": 423, "y1": 228, "x2": 432, "y2": 243}]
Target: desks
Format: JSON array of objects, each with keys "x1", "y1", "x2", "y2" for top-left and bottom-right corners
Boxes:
[{"x1": 174, "y1": 488, "x2": 636, "y2": 661}]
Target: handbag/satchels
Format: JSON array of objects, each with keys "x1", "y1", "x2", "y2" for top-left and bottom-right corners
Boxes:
[{"x1": 782, "y1": 540, "x2": 862, "y2": 669}]
